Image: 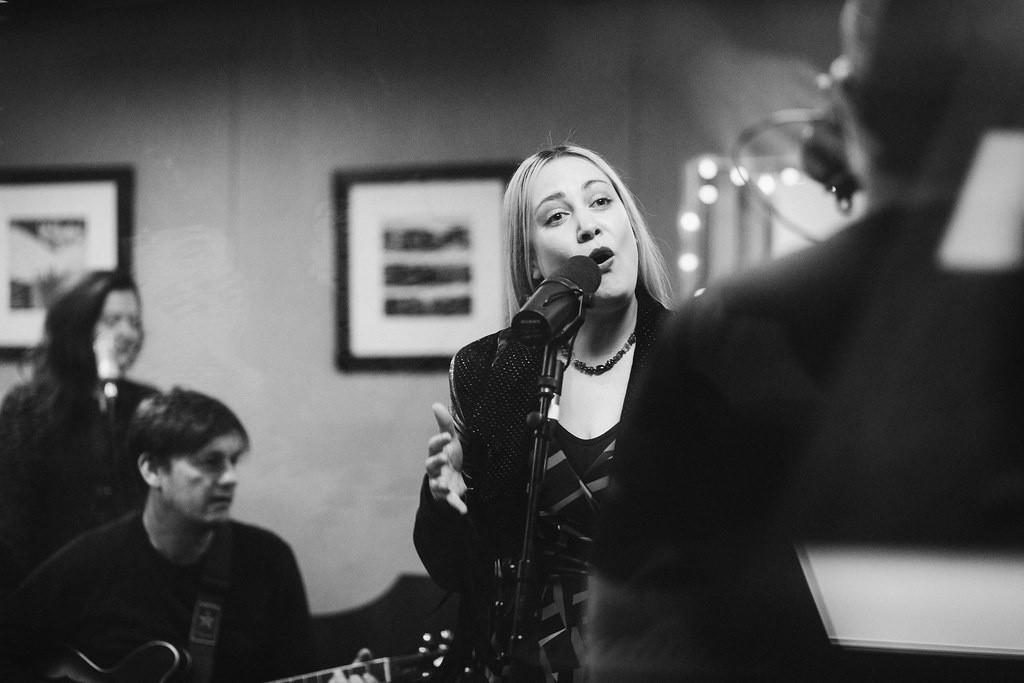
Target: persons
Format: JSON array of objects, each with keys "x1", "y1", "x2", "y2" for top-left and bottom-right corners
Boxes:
[
  {"x1": 0, "y1": 271, "x2": 380, "y2": 683},
  {"x1": 413, "y1": 1, "x2": 1024, "y2": 683}
]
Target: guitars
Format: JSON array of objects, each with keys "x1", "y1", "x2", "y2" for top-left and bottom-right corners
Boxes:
[{"x1": 39, "y1": 629, "x2": 495, "y2": 683}]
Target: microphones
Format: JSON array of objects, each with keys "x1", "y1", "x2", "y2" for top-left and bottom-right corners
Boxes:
[{"x1": 509, "y1": 255, "x2": 602, "y2": 347}]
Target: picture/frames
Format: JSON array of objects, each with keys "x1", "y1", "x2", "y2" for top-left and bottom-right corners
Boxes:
[
  {"x1": 329, "y1": 161, "x2": 523, "y2": 372},
  {"x1": 0, "y1": 163, "x2": 134, "y2": 364}
]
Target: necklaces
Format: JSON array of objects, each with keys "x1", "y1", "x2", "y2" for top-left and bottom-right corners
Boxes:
[{"x1": 560, "y1": 333, "x2": 636, "y2": 375}]
